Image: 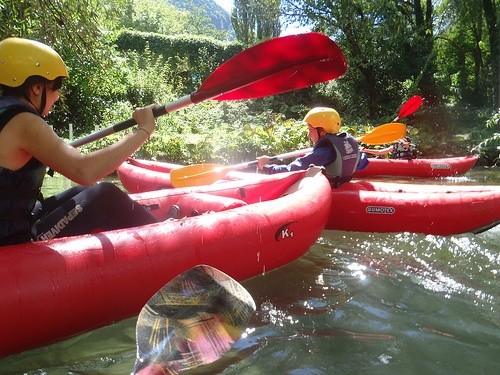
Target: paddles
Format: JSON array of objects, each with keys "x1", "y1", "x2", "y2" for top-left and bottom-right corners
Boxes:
[
  {"x1": 390, "y1": 94, "x2": 422, "y2": 123},
  {"x1": 170, "y1": 122, "x2": 406, "y2": 189},
  {"x1": 69, "y1": 32, "x2": 348, "y2": 149}
]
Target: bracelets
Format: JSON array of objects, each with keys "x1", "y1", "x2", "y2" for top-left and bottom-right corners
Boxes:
[{"x1": 139, "y1": 128, "x2": 150, "y2": 136}]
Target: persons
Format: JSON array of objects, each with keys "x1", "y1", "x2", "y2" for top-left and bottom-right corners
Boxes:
[
  {"x1": 360, "y1": 133, "x2": 424, "y2": 160},
  {"x1": 256, "y1": 107, "x2": 361, "y2": 188},
  {"x1": 0, "y1": 34, "x2": 181, "y2": 246}
]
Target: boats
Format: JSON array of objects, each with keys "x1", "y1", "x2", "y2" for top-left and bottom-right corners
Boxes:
[
  {"x1": 0, "y1": 164, "x2": 334, "y2": 359},
  {"x1": 297, "y1": 154, "x2": 481, "y2": 179},
  {"x1": 117, "y1": 158, "x2": 500, "y2": 237}
]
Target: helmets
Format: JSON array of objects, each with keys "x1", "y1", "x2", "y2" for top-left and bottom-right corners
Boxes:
[
  {"x1": 304, "y1": 107, "x2": 342, "y2": 134},
  {"x1": 0, "y1": 37, "x2": 69, "y2": 87}
]
